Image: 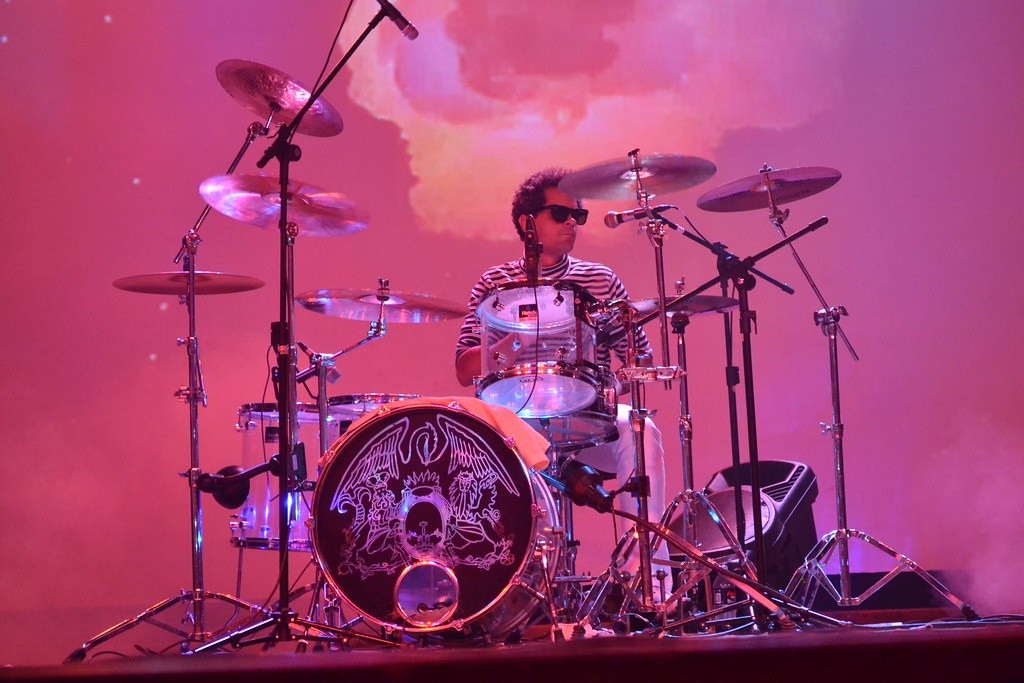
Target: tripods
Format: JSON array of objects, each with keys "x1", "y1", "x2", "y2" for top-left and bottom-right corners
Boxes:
[
  {"x1": 569, "y1": 184, "x2": 982, "y2": 639},
  {"x1": 60, "y1": 13, "x2": 416, "y2": 666}
]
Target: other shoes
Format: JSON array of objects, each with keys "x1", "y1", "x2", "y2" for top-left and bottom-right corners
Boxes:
[{"x1": 669, "y1": 596, "x2": 691, "y2": 618}]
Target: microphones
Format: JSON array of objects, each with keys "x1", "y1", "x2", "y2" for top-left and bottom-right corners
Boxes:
[
  {"x1": 595, "y1": 313, "x2": 623, "y2": 347},
  {"x1": 524, "y1": 217, "x2": 539, "y2": 289},
  {"x1": 604, "y1": 205, "x2": 671, "y2": 228},
  {"x1": 298, "y1": 343, "x2": 340, "y2": 383},
  {"x1": 376, "y1": 0, "x2": 419, "y2": 40}
]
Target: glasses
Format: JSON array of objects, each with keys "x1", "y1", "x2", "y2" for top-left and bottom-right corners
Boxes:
[{"x1": 538, "y1": 205, "x2": 588, "y2": 225}]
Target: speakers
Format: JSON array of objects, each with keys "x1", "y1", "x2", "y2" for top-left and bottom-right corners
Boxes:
[{"x1": 669, "y1": 460, "x2": 818, "y2": 630}]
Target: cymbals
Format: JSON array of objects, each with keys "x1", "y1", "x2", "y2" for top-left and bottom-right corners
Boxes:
[
  {"x1": 558, "y1": 153, "x2": 716, "y2": 198},
  {"x1": 294, "y1": 288, "x2": 471, "y2": 324},
  {"x1": 217, "y1": 59, "x2": 343, "y2": 137},
  {"x1": 632, "y1": 294, "x2": 740, "y2": 315},
  {"x1": 199, "y1": 176, "x2": 368, "y2": 237},
  {"x1": 114, "y1": 272, "x2": 265, "y2": 295},
  {"x1": 696, "y1": 167, "x2": 841, "y2": 212}
]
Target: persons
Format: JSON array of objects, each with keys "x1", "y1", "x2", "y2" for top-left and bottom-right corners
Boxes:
[{"x1": 455, "y1": 167, "x2": 692, "y2": 614}]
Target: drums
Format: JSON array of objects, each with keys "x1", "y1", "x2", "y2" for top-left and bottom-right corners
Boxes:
[
  {"x1": 475, "y1": 278, "x2": 598, "y2": 419},
  {"x1": 323, "y1": 395, "x2": 436, "y2": 448},
  {"x1": 232, "y1": 403, "x2": 360, "y2": 551},
  {"x1": 310, "y1": 396, "x2": 563, "y2": 641},
  {"x1": 523, "y1": 366, "x2": 619, "y2": 455}
]
